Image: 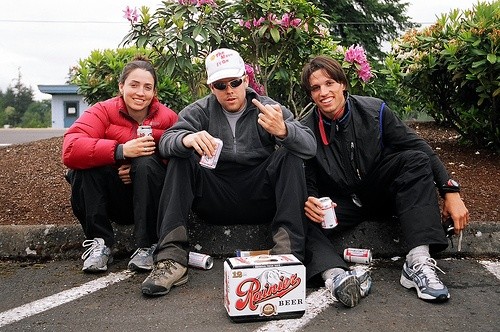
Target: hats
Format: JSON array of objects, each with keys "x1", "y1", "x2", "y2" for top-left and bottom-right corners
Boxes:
[{"x1": 205, "y1": 49, "x2": 246, "y2": 85}]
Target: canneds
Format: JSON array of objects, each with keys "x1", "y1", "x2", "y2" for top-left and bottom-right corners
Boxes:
[
  {"x1": 343, "y1": 247, "x2": 372, "y2": 264},
  {"x1": 137, "y1": 126, "x2": 152, "y2": 148},
  {"x1": 316, "y1": 197, "x2": 338, "y2": 229},
  {"x1": 199, "y1": 138, "x2": 223, "y2": 169},
  {"x1": 188, "y1": 251, "x2": 213, "y2": 270}
]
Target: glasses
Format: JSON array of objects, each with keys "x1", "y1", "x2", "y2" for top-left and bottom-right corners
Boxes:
[{"x1": 211, "y1": 75, "x2": 246, "y2": 90}]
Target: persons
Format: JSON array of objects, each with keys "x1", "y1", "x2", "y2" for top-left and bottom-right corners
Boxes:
[
  {"x1": 299, "y1": 56, "x2": 470, "y2": 307},
  {"x1": 142, "y1": 48, "x2": 316, "y2": 297},
  {"x1": 62, "y1": 55, "x2": 179, "y2": 272}
]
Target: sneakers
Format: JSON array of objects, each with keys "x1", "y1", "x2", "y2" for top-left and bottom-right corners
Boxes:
[
  {"x1": 400, "y1": 255, "x2": 450, "y2": 299},
  {"x1": 141, "y1": 260, "x2": 188, "y2": 296},
  {"x1": 81, "y1": 238, "x2": 113, "y2": 271},
  {"x1": 128, "y1": 244, "x2": 159, "y2": 272},
  {"x1": 324, "y1": 266, "x2": 372, "y2": 308}
]
